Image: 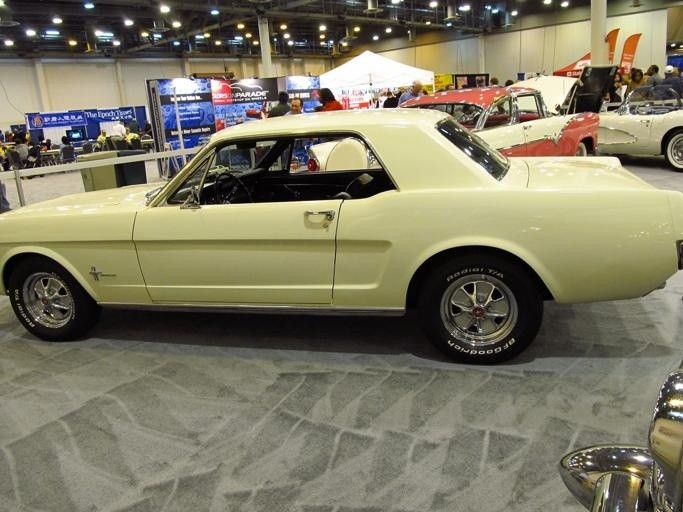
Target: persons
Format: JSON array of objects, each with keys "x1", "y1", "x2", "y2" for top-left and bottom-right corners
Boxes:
[
  {"x1": 314, "y1": 88, "x2": 344, "y2": 111},
  {"x1": 0, "y1": 117, "x2": 153, "y2": 174},
  {"x1": 303, "y1": 90, "x2": 321, "y2": 112},
  {"x1": 236, "y1": 118, "x2": 256, "y2": 168},
  {"x1": 267, "y1": 92, "x2": 290, "y2": 117},
  {"x1": 603, "y1": 64, "x2": 683, "y2": 110},
  {"x1": 366, "y1": 77, "x2": 512, "y2": 108},
  {"x1": 284, "y1": 98, "x2": 307, "y2": 115}
]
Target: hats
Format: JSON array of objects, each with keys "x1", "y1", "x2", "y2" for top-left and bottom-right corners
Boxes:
[{"x1": 665, "y1": 65, "x2": 674, "y2": 74}]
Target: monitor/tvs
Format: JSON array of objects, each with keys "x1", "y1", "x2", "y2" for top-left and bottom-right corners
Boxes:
[{"x1": 66, "y1": 129, "x2": 84, "y2": 141}]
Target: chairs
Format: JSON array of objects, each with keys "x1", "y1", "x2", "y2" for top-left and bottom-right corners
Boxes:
[
  {"x1": 0, "y1": 134, "x2": 155, "y2": 179},
  {"x1": 344, "y1": 172, "x2": 373, "y2": 199},
  {"x1": 460, "y1": 112, "x2": 539, "y2": 129}
]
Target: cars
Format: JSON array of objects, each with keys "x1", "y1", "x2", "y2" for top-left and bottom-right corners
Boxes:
[
  {"x1": 0, "y1": 106, "x2": 683, "y2": 363},
  {"x1": 597, "y1": 85, "x2": 683, "y2": 171},
  {"x1": 397, "y1": 75, "x2": 600, "y2": 156}
]
